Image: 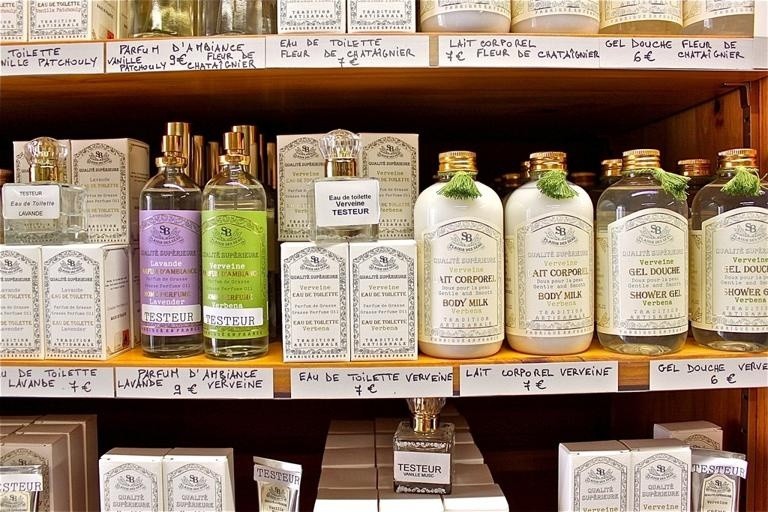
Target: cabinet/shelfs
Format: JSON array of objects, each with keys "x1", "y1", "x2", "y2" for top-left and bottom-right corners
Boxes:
[{"x1": 0, "y1": 33, "x2": 768, "y2": 511}]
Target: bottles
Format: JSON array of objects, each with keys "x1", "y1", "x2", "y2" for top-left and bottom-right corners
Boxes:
[
  {"x1": 3, "y1": 121, "x2": 768, "y2": 360},
  {"x1": 3, "y1": 2, "x2": 768, "y2": 42}
]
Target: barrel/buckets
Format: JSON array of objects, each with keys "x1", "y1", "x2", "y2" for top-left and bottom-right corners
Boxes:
[{"x1": 253, "y1": 456, "x2": 303, "y2": 512}]
